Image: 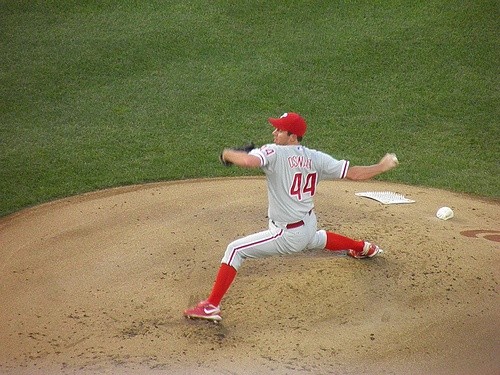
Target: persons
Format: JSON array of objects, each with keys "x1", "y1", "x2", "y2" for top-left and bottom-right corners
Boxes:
[{"x1": 184, "y1": 113, "x2": 399, "y2": 320}]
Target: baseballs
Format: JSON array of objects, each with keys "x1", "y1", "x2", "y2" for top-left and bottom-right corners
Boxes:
[{"x1": 392, "y1": 154, "x2": 398, "y2": 161}]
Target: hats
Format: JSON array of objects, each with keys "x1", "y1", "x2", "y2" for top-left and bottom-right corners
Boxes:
[{"x1": 269, "y1": 112, "x2": 307, "y2": 136}]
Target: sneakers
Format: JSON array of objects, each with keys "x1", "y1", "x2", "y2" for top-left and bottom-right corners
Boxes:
[
  {"x1": 348, "y1": 241, "x2": 379, "y2": 257},
  {"x1": 184, "y1": 300, "x2": 222, "y2": 320}
]
